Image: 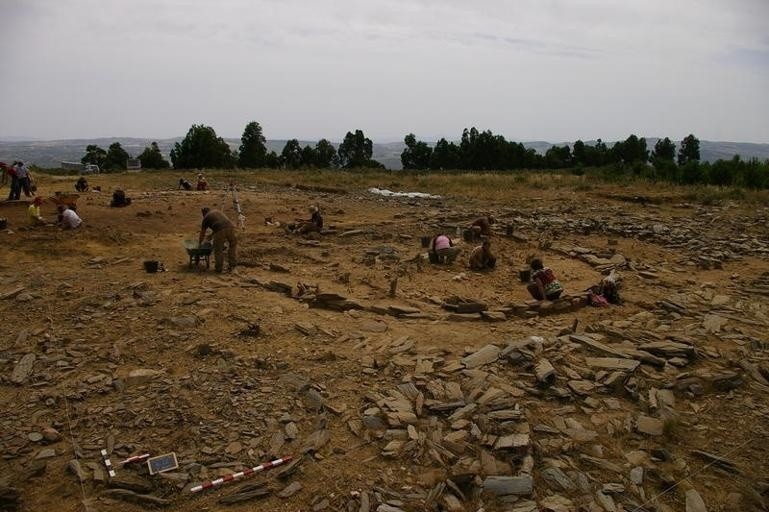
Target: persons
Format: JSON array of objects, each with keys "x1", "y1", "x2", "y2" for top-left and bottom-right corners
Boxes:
[
  {"x1": 74, "y1": 176, "x2": 90, "y2": 192},
  {"x1": 56, "y1": 205, "x2": 82, "y2": 229},
  {"x1": 469, "y1": 214, "x2": 501, "y2": 243},
  {"x1": 468, "y1": 242, "x2": 498, "y2": 270},
  {"x1": 27, "y1": 197, "x2": 48, "y2": 227},
  {"x1": 431, "y1": 232, "x2": 462, "y2": 265},
  {"x1": 197, "y1": 173, "x2": 210, "y2": 191},
  {"x1": 527, "y1": 259, "x2": 563, "y2": 305},
  {"x1": 7, "y1": 159, "x2": 35, "y2": 200},
  {"x1": 178, "y1": 178, "x2": 193, "y2": 190},
  {"x1": 296, "y1": 205, "x2": 323, "y2": 234},
  {"x1": 197, "y1": 207, "x2": 238, "y2": 274}
]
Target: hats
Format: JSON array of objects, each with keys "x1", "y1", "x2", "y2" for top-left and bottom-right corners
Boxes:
[
  {"x1": 35, "y1": 198, "x2": 42, "y2": 205},
  {"x1": 308, "y1": 205, "x2": 318, "y2": 214}
]
